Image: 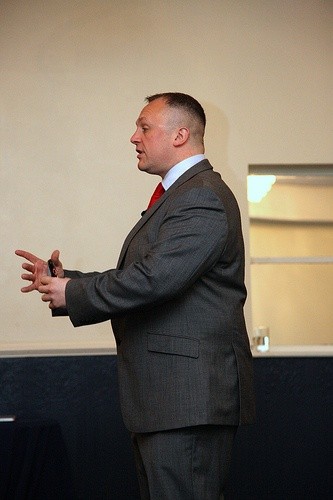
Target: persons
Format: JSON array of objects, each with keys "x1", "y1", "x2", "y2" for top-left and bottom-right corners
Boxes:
[{"x1": 12, "y1": 91, "x2": 258, "y2": 500}]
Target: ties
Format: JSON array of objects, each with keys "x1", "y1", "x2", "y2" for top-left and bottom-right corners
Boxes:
[{"x1": 147, "y1": 182, "x2": 164, "y2": 210}]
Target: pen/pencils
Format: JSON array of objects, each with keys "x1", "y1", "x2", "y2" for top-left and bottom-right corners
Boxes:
[{"x1": 48, "y1": 259, "x2": 57, "y2": 277}]
